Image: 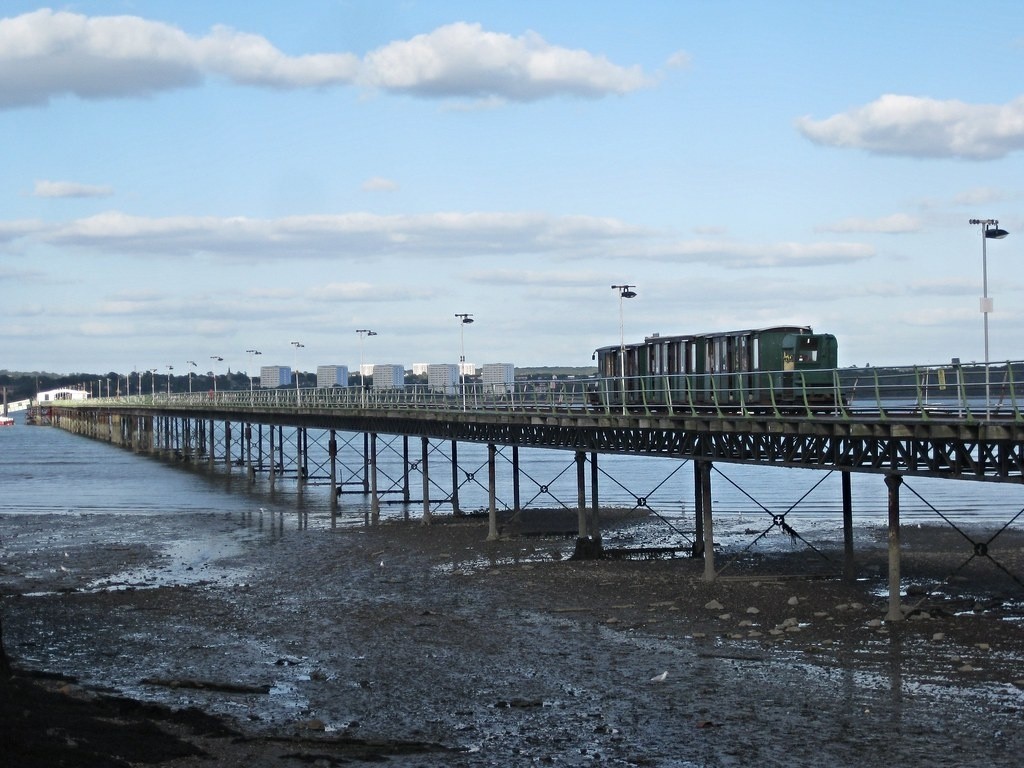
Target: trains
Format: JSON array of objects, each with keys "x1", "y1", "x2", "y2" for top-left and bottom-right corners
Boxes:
[{"x1": 591, "y1": 325, "x2": 849, "y2": 413}]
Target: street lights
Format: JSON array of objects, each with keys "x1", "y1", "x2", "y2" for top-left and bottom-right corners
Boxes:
[
  {"x1": 245, "y1": 349, "x2": 262, "y2": 405},
  {"x1": 186, "y1": 360, "x2": 198, "y2": 397},
  {"x1": 968, "y1": 219, "x2": 1011, "y2": 422},
  {"x1": 454, "y1": 314, "x2": 474, "y2": 413},
  {"x1": 149, "y1": 368, "x2": 157, "y2": 405},
  {"x1": 355, "y1": 328, "x2": 378, "y2": 408},
  {"x1": 165, "y1": 365, "x2": 175, "y2": 398},
  {"x1": 291, "y1": 341, "x2": 304, "y2": 408},
  {"x1": 210, "y1": 356, "x2": 224, "y2": 405},
  {"x1": 611, "y1": 284, "x2": 638, "y2": 417}
]
